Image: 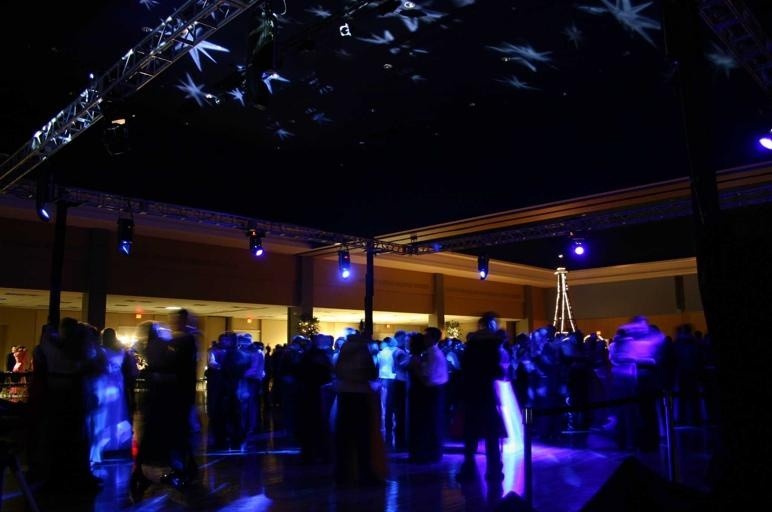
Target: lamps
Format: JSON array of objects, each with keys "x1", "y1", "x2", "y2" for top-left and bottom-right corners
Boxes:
[
  {"x1": 247, "y1": 220, "x2": 265, "y2": 257},
  {"x1": 117, "y1": 199, "x2": 134, "y2": 256},
  {"x1": 110, "y1": 88, "x2": 126, "y2": 126},
  {"x1": 35, "y1": 159, "x2": 53, "y2": 221},
  {"x1": 478, "y1": 246, "x2": 488, "y2": 280},
  {"x1": 338, "y1": 243, "x2": 353, "y2": 279}
]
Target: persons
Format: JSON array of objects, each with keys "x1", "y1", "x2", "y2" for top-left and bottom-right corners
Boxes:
[{"x1": 0, "y1": 310, "x2": 710, "y2": 512}]
